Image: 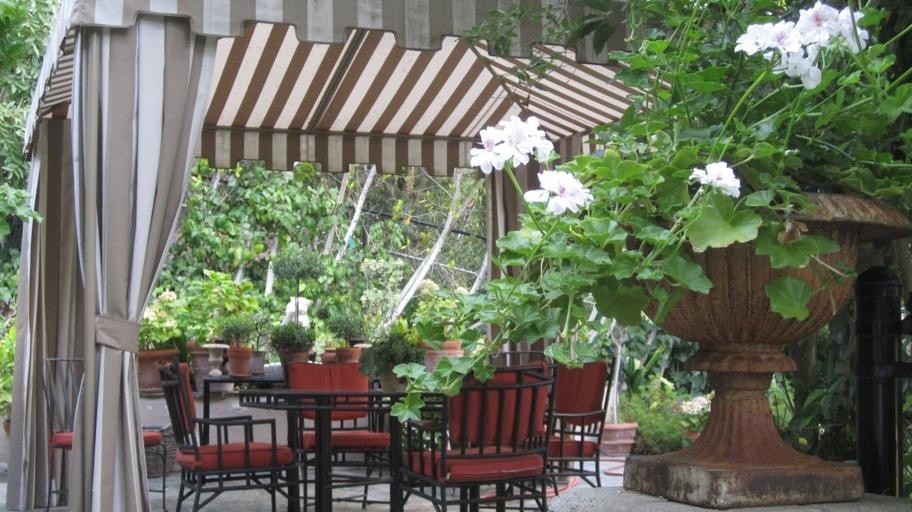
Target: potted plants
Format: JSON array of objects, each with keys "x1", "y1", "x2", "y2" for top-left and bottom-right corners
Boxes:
[{"x1": 185, "y1": 241, "x2": 471, "y2": 399}]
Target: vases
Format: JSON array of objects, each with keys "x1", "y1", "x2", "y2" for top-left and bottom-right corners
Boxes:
[
  {"x1": 385, "y1": 1, "x2": 912, "y2": 445},
  {"x1": 134, "y1": 290, "x2": 180, "y2": 351},
  {"x1": 597, "y1": 191, "x2": 912, "y2": 511},
  {"x1": 131, "y1": 346, "x2": 183, "y2": 399}
]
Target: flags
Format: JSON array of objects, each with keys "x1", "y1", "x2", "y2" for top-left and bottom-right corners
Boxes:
[
  {"x1": 131, "y1": 346, "x2": 183, "y2": 399},
  {"x1": 597, "y1": 191, "x2": 912, "y2": 511}
]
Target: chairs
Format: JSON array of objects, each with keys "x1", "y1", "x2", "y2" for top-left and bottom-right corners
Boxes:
[{"x1": 38, "y1": 349, "x2": 614, "y2": 512}]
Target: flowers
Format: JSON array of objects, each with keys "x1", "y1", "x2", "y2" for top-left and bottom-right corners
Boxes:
[
  {"x1": 385, "y1": 1, "x2": 912, "y2": 445},
  {"x1": 134, "y1": 290, "x2": 180, "y2": 351}
]
